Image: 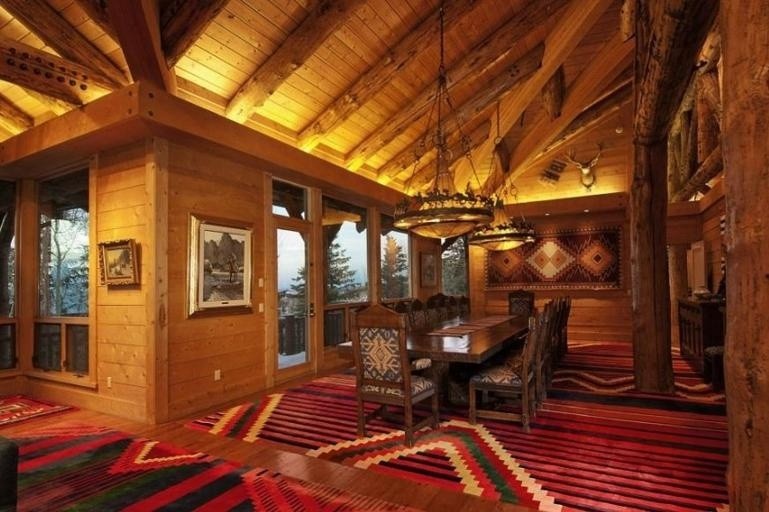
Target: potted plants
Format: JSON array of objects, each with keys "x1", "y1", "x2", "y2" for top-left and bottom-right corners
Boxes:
[
  {"x1": 97, "y1": 236, "x2": 139, "y2": 290},
  {"x1": 188, "y1": 209, "x2": 258, "y2": 320}
]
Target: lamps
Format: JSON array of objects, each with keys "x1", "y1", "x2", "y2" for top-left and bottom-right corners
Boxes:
[{"x1": 351, "y1": 289, "x2": 572, "y2": 449}]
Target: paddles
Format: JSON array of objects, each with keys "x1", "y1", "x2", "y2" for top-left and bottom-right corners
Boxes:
[
  {"x1": 0, "y1": 389, "x2": 81, "y2": 428},
  {"x1": 9, "y1": 423, "x2": 428, "y2": 512},
  {"x1": 182, "y1": 338, "x2": 730, "y2": 512}
]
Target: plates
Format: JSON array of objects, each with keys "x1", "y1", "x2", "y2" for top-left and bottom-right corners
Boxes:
[{"x1": 675, "y1": 291, "x2": 722, "y2": 371}]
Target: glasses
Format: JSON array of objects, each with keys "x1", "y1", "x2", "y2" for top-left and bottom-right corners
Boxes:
[
  {"x1": 470, "y1": 122, "x2": 538, "y2": 253},
  {"x1": 390, "y1": 3, "x2": 498, "y2": 245}
]
